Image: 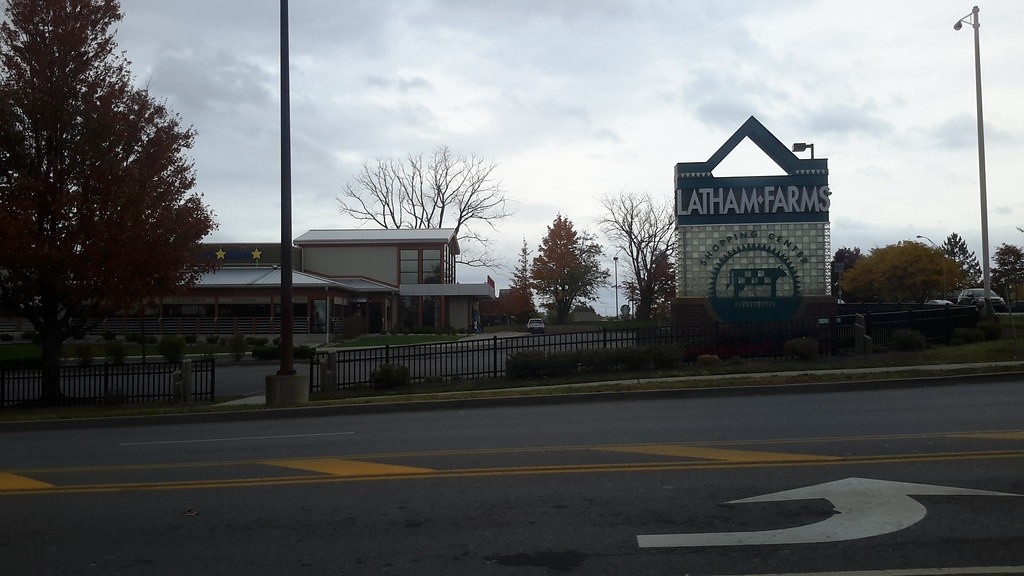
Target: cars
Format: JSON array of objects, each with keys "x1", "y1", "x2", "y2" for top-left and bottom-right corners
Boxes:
[{"x1": 928, "y1": 300, "x2": 954, "y2": 306}]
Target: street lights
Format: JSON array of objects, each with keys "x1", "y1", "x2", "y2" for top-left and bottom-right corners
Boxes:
[
  {"x1": 951, "y1": 7, "x2": 999, "y2": 316},
  {"x1": 792, "y1": 142, "x2": 816, "y2": 159},
  {"x1": 917, "y1": 235, "x2": 948, "y2": 300},
  {"x1": 613, "y1": 255, "x2": 625, "y2": 323}
]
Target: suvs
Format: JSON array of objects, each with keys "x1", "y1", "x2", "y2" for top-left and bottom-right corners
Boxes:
[
  {"x1": 528, "y1": 317, "x2": 547, "y2": 333},
  {"x1": 956, "y1": 287, "x2": 1007, "y2": 313}
]
